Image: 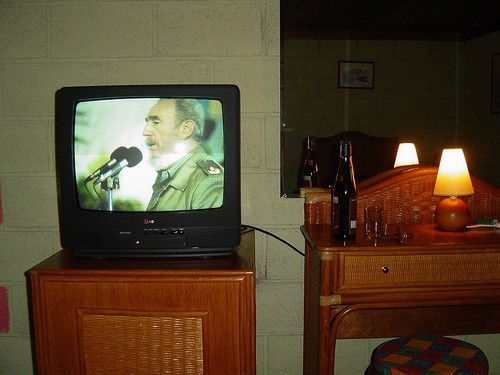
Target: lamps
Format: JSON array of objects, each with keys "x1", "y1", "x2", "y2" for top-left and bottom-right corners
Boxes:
[
  {"x1": 394, "y1": 142, "x2": 419, "y2": 169},
  {"x1": 433, "y1": 146, "x2": 476, "y2": 231}
]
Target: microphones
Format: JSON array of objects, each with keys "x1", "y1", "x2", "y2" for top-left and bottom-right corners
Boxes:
[
  {"x1": 94, "y1": 147, "x2": 143, "y2": 184},
  {"x1": 85, "y1": 147, "x2": 128, "y2": 182}
]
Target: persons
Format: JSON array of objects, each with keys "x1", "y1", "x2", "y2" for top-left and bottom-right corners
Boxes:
[{"x1": 142, "y1": 97, "x2": 224, "y2": 209}]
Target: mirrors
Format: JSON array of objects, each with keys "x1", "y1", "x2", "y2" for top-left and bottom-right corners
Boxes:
[{"x1": 280, "y1": 0, "x2": 500, "y2": 199}]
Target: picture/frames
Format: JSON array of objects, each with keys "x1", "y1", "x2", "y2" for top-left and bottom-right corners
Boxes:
[{"x1": 338, "y1": 61, "x2": 376, "y2": 89}]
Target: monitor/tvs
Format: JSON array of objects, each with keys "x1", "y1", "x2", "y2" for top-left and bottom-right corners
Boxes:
[{"x1": 55, "y1": 84, "x2": 241, "y2": 259}]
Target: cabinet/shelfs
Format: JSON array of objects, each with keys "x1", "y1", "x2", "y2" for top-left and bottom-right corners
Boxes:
[{"x1": 300, "y1": 168, "x2": 500, "y2": 375}]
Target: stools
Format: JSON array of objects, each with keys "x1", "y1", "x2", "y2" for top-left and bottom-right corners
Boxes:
[{"x1": 365, "y1": 336, "x2": 490, "y2": 375}]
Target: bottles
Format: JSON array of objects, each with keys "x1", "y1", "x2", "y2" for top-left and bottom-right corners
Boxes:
[
  {"x1": 331, "y1": 139, "x2": 358, "y2": 240},
  {"x1": 299, "y1": 136, "x2": 320, "y2": 189}
]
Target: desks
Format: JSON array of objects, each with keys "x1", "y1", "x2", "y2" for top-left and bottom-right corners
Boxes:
[{"x1": 23, "y1": 251, "x2": 256, "y2": 375}]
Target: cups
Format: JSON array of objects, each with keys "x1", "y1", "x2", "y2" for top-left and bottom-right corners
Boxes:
[{"x1": 365, "y1": 205, "x2": 386, "y2": 242}]
